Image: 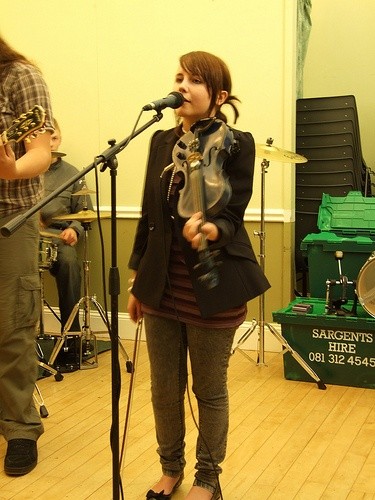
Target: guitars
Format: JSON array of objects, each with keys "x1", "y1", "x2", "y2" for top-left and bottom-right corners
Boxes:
[{"x1": 1, "y1": 104, "x2": 46, "y2": 145}]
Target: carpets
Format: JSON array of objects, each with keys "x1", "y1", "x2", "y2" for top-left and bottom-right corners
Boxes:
[{"x1": 37, "y1": 337, "x2": 111, "y2": 381}]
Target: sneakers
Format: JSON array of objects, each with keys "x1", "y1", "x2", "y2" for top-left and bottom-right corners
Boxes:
[{"x1": 4, "y1": 438, "x2": 38, "y2": 476}]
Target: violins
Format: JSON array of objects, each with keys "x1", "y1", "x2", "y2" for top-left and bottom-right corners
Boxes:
[{"x1": 172, "y1": 118, "x2": 234, "y2": 292}]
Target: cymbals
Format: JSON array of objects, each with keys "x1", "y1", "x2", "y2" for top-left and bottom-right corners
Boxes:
[
  {"x1": 50, "y1": 210, "x2": 118, "y2": 220},
  {"x1": 255, "y1": 143, "x2": 308, "y2": 164},
  {"x1": 70, "y1": 189, "x2": 98, "y2": 196}
]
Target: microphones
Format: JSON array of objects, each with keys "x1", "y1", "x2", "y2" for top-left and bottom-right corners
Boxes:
[{"x1": 142, "y1": 91, "x2": 185, "y2": 110}]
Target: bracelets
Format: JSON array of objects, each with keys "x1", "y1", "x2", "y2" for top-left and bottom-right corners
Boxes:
[{"x1": 128, "y1": 279, "x2": 134, "y2": 293}]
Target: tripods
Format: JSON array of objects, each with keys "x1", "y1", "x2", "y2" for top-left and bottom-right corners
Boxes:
[
  {"x1": 227, "y1": 156, "x2": 326, "y2": 389},
  {"x1": 40, "y1": 220, "x2": 135, "y2": 378}
]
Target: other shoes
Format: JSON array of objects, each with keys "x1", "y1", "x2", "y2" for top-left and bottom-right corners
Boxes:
[
  {"x1": 62, "y1": 337, "x2": 94, "y2": 356},
  {"x1": 211, "y1": 489, "x2": 221, "y2": 500},
  {"x1": 146, "y1": 472, "x2": 184, "y2": 500}
]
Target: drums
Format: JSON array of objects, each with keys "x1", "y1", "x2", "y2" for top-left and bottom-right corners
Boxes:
[
  {"x1": 355, "y1": 251, "x2": 375, "y2": 318},
  {"x1": 37, "y1": 237, "x2": 58, "y2": 267}
]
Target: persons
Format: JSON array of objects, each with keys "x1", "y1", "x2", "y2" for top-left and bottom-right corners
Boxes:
[
  {"x1": 126, "y1": 51, "x2": 270, "y2": 499},
  {"x1": 40, "y1": 119, "x2": 94, "y2": 365},
  {"x1": 0, "y1": 36, "x2": 55, "y2": 474}
]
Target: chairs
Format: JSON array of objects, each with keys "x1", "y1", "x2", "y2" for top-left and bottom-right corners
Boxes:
[{"x1": 296, "y1": 95, "x2": 371, "y2": 297}]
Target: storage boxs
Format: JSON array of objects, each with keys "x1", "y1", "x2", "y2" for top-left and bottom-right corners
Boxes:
[{"x1": 272, "y1": 297, "x2": 375, "y2": 389}]
